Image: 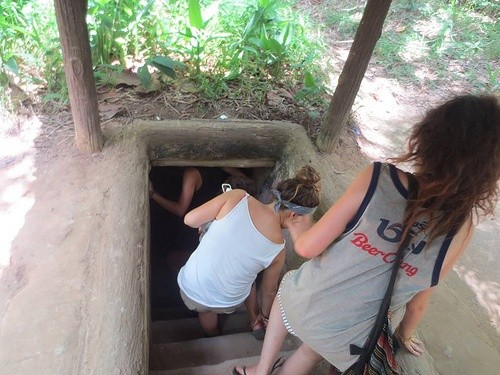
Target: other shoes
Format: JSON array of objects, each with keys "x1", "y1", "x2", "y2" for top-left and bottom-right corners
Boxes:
[
  {"x1": 249, "y1": 313, "x2": 266, "y2": 341},
  {"x1": 231, "y1": 363, "x2": 248, "y2": 375},
  {"x1": 269, "y1": 355, "x2": 286, "y2": 375}
]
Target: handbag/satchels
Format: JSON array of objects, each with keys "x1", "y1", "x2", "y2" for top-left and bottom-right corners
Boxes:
[{"x1": 340, "y1": 315, "x2": 404, "y2": 375}]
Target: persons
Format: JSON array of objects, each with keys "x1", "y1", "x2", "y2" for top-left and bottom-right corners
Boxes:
[
  {"x1": 234, "y1": 93, "x2": 500, "y2": 375},
  {"x1": 177, "y1": 166, "x2": 322, "y2": 339},
  {"x1": 149, "y1": 167, "x2": 256, "y2": 264}
]
